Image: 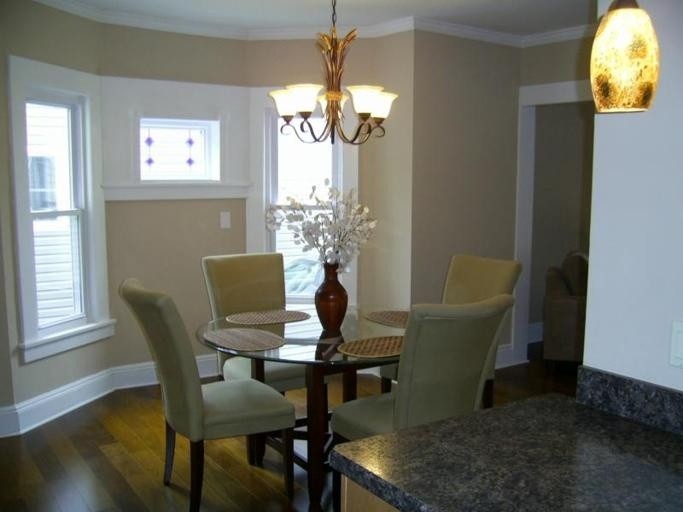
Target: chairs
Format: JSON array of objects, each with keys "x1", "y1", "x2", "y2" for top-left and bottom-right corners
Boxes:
[
  {"x1": 200, "y1": 251, "x2": 316, "y2": 399},
  {"x1": 330, "y1": 293, "x2": 515, "y2": 509},
  {"x1": 117, "y1": 275, "x2": 295, "y2": 510},
  {"x1": 378, "y1": 251, "x2": 525, "y2": 407}
]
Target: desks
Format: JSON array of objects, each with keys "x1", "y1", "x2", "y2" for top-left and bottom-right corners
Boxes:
[{"x1": 192, "y1": 298, "x2": 414, "y2": 511}]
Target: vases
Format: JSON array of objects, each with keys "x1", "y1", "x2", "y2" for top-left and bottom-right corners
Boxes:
[{"x1": 314, "y1": 262, "x2": 347, "y2": 330}]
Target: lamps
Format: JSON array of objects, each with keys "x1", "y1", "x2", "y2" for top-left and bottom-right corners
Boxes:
[
  {"x1": 583, "y1": 0, "x2": 658, "y2": 115},
  {"x1": 265, "y1": 0, "x2": 399, "y2": 144}
]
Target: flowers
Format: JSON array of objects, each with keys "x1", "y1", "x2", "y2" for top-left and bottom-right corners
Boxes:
[{"x1": 265, "y1": 179, "x2": 376, "y2": 273}]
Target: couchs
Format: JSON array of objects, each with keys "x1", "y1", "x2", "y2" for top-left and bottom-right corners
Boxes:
[{"x1": 540, "y1": 248, "x2": 588, "y2": 365}]
